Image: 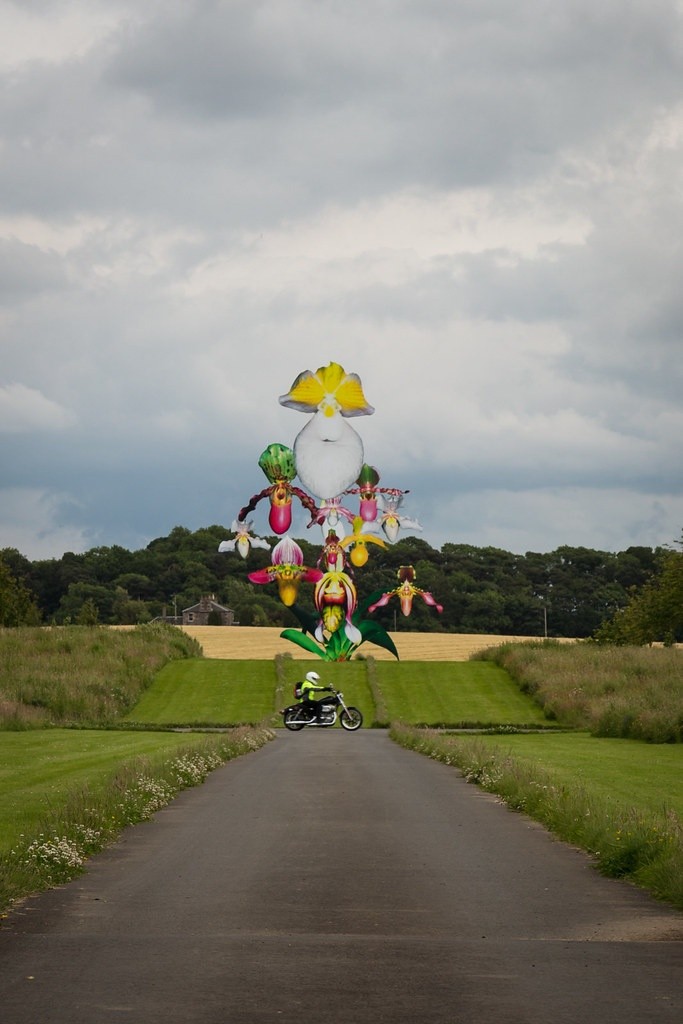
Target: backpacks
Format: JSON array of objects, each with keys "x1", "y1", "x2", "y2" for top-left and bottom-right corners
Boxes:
[{"x1": 293, "y1": 682, "x2": 309, "y2": 699}]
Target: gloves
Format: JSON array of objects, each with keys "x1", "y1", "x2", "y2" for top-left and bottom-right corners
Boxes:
[{"x1": 322, "y1": 686, "x2": 333, "y2": 692}]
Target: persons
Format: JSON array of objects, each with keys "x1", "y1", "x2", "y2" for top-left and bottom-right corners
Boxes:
[{"x1": 300, "y1": 672, "x2": 333, "y2": 723}]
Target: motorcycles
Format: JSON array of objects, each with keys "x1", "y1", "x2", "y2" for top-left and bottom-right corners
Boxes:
[{"x1": 279, "y1": 683, "x2": 363, "y2": 731}]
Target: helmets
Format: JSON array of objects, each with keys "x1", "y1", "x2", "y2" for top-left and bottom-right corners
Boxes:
[{"x1": 306, "y1": 671, "x2": 320, "y2": 685}]
